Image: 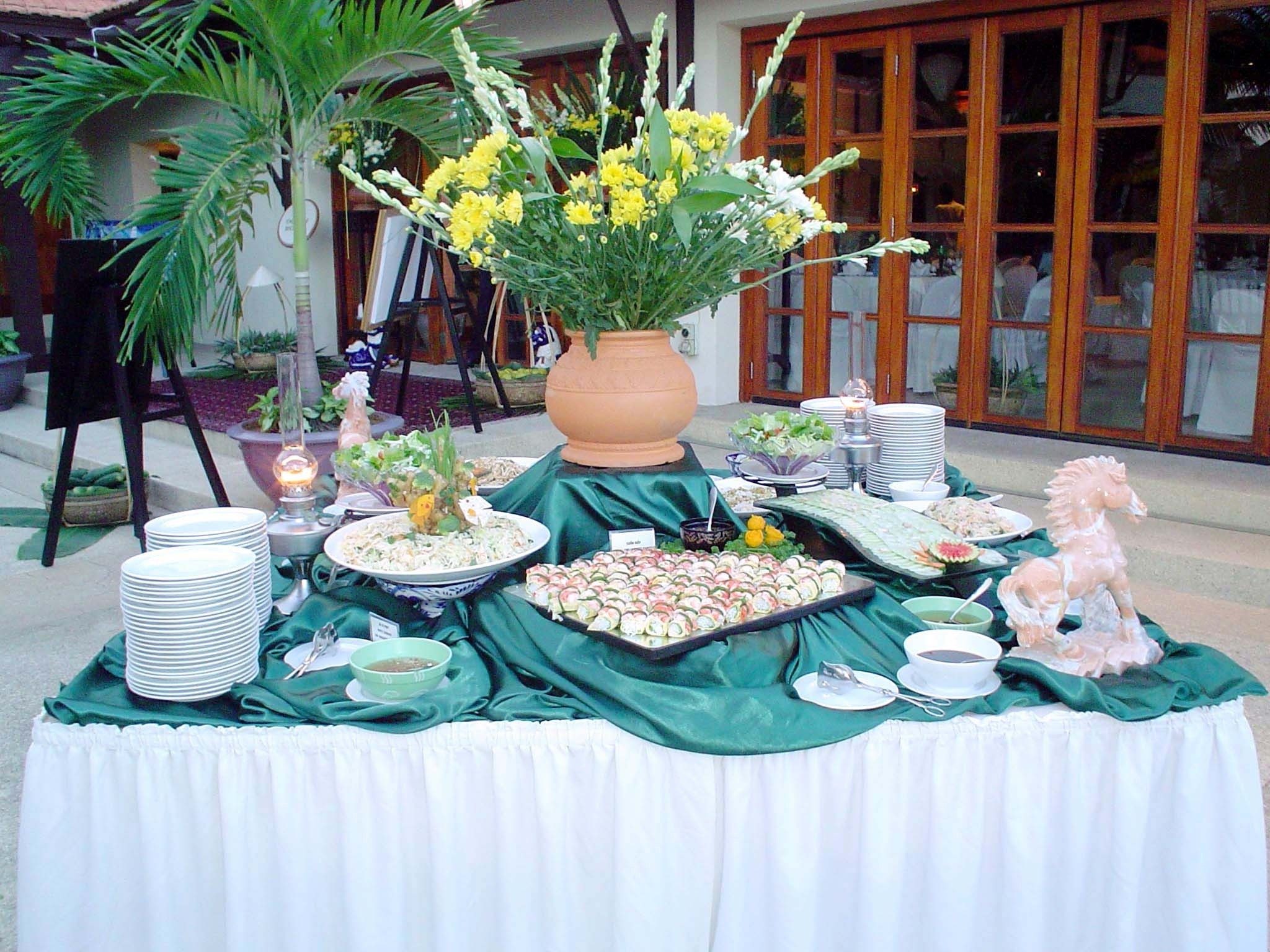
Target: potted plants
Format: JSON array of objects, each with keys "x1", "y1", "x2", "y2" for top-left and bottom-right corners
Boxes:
[
  {"x1": 933, "y1": 355, "x2": 1044, "y2": 417},
  {"x1": 0, "y1": 330, "x2": 33, "y2": 412},
  {"x1": 228, "y1": 376, "x2": 405, "y2": 506},
  {"x1": 215, "y1": 329, "x2": 298, "y2": 371}
]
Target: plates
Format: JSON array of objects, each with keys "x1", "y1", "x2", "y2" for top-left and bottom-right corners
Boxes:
[
  {"x1": 335, "y1": 491, "x2": 409, "y2": 515},
  {"x1": 345, "y1": 674, "x2": 450, "y2": 704},
  {"x1": 800, "y1": 397, "x2": 946, "y2": 497},
  {"x1": 459, "y1": 456, "x2": 540, "y2": 495},
  {"x1": 792, "y1": 670, "x2": 899, "y2": 711},
  {"x1": 323, "y1": 505, "x2": 364, "y2": 515},
  {"x1": 739, "y1": 459, "x2": 830, "y2": 483},
  {"x1": 119, "y1": 505, "x2": 274, "y2": 704},
  {"x1": 714, "y1": 476, "x2": 827, "y2": 519},
  {"x1": 897, "y1": 662, "x2": 1001, "y2": 700},
  {"x1": 284, "y1": 637, "x2": 374, "y2": 672},
  {"x1": 323, "y1": 511, "x2": 551, "y2": 583}
]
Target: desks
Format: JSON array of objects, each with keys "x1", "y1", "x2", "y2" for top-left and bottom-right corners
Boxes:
[
  {"x1": 823, "y1": 274, "x2": 943, "y2": 356},
  {"x1": 14, "y1": 554, "x2": 1267, "y2": 952}
]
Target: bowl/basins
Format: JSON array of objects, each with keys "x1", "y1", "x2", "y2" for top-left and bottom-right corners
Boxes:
[
  {"x1": 679, "y1": 516, "x2": 738, "y2": 555},
  {"x1": 888, "y1": 481, "x2": 951, "y2": 502},
  {"x1": 903, "y1": 629, "x2": 1002, "y2": 690},
  {"x1": 890, "y1": 500, "x2": 1033, "y2": 547},
  {"x1": 348, "y1": 637, "x2": 453, "y2": 700},
  {"x1": 725, "y1": 453, "x2": 747, "y2": 478},
  {"x1": 901, "y1": 596, "x2": 994, "y2": 634}
]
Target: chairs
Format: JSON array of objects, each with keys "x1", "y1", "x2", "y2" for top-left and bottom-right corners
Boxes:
[{"x1": 768, "y1": 254, "x2": 1264, "y2": 437}]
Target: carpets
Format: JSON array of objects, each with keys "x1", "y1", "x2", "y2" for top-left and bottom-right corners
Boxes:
[{"x1": 146, "y1": 368, "x2": 546, "y2": 431}]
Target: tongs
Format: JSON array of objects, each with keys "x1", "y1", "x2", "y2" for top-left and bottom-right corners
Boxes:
[
  {"x1": 282, "y1": 621, "x2": 339, "y2": 680},
  {"x1": 817, "y1": 660, "x2": 952, "y2": 717}
]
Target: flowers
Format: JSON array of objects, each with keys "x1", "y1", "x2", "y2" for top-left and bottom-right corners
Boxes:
[{"x1": 335, "y1": 12, "x2": 930, "y2": 357}]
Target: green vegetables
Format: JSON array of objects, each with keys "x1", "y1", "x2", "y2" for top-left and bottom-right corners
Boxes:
[
  {"x1": 730, "y1": 409, "x2": 834, "y2": 454},
  {"x1": 330, "y1": 429, "x2": 534, "y2": 566}
]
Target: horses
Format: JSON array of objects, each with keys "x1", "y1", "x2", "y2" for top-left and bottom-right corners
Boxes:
[
  {"x1": 996, "y1": 450, "x2": 1151, "y2": 662},
  {"x1": 330, "y1": 368, "x2": 376, "y2": 498}
]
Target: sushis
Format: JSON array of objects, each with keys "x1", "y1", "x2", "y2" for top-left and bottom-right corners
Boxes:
[{"x1": 524, "y1": 547, "x2": 844, "y2": 637}]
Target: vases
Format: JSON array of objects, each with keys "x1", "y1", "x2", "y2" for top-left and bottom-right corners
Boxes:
[{"x1": 544, "y1": 329, "x2": 697, "y2": 468}]
